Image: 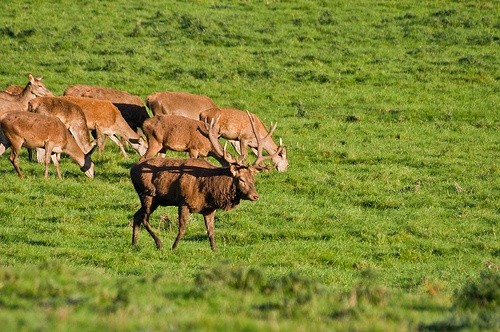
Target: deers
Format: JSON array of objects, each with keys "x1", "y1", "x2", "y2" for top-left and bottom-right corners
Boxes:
[
  {"x1": 131, "y1": 109, "x2": 284, "y2": 250},
  {"x1": 0, "y1": 74, "x2": 55, "y2": 156},
  {"x1": 56, "y1": 95, "x2": 149, "y2": 161},
  {"x1": 199, "y1": 107, "x2": 290, "y2": 174},
  {"x1": 136, "y1": 115, "x2": 246, "y2": 167},
  {"x1": 146, "y1": 92, "x2": 219, "y2": 121},
  {"x1": 0, "y1": 111, "x2": 95, "y2": 180},
  {"x1": 28, "y1": 96, "x2": 101, "y2": 161},
  {"x1": 63, "y1": 86, "x2": 151, "y2": 152}
]
